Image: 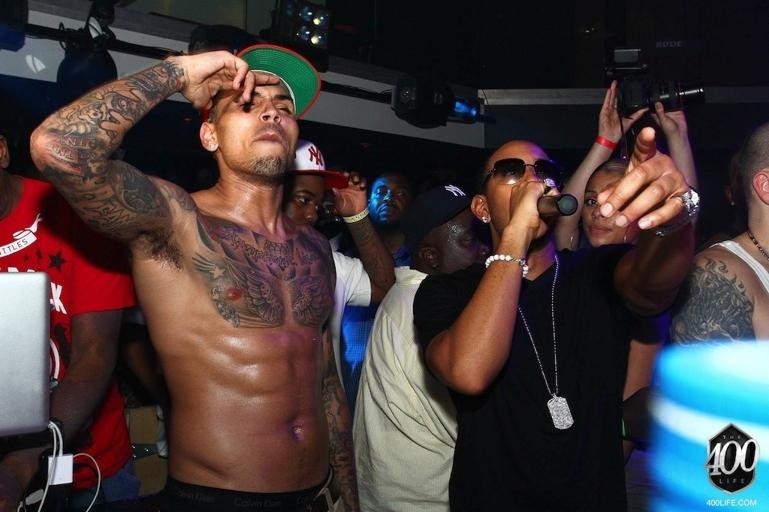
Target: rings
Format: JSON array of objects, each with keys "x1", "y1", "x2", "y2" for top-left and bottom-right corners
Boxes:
[{"x1": 680, "y1": 189, "x2": 703, "y2": 217}]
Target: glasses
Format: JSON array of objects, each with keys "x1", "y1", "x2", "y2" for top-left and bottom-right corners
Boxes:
[{"x1": 477, "y1": 158, "x2": 563, "y2": 195}]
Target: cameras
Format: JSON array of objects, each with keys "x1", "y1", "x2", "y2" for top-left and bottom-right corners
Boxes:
[{"x1": 605, "y1": 44, "x2": 706, "y2": 117}]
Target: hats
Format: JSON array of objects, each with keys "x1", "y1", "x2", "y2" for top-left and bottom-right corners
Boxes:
[
  {"x1": 404, "y1": 182, "x2": 471, "y2": 250},
  {"x1": 287, "y1": 137, "x2": 348, "y2": 194},
  {"x1": 201, "y1": 44, "x2": 321, "y2": 132}
]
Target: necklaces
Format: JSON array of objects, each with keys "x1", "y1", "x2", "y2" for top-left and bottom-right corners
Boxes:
[
  {"x1": 743, "y1": 222, "x2": 769, "y2": 262},
  {"x1": 486, "y1": 250, "x2": 579, "y2": 432}
]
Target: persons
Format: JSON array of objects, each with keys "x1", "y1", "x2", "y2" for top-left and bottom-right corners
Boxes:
[
  {"x1": 0, "y1": 125, "x2": 142, "y2": 510},
  {"x1": 27, "y1": 41, "x2": 361, "y2": 511},
  {"x1": 411, "y1": 125, "x2": 702, "y2": 512},
  {"x1": 279, "y1": 135, "x2": 401, "y2": 383},
  {"x1": 665, "y1": 120, "x2": 768, "y2": 340},
  {"x1": 52, "y1": 309, "x2": 173, "y2": 509},
  {"x1": 556, "y1": 75, "x2": 704, "y2": 462},
  {"x1": 338, "y1": 170, "x2": 411, "y2": 424},
  {"x1": 332, "y1": 182, "x2": 493, "y2": 512}
]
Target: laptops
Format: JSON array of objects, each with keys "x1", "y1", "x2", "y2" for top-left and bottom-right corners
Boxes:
[{"x1": 0, "y1": 271, "x2": 49, "y2": 438}]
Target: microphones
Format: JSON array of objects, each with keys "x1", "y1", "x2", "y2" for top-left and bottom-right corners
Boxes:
[{"x1": 537, "y1": 194, "x2": 577, "y2": 221}]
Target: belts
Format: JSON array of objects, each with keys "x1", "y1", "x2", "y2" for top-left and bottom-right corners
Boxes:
[{"x1": 310, "y1": 453, "x2": 344, "y2": 509}]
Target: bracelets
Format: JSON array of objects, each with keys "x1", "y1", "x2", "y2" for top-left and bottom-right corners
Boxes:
[
  {"x1": 597, "y1": 135, "x2": 619, "y2": 152},
  {"x1": 340, "y1": 205, "x2": 373, "y2": 226},
  {"x1": 483, "y1": 252, "x2": 529, "y2": 282}
]
